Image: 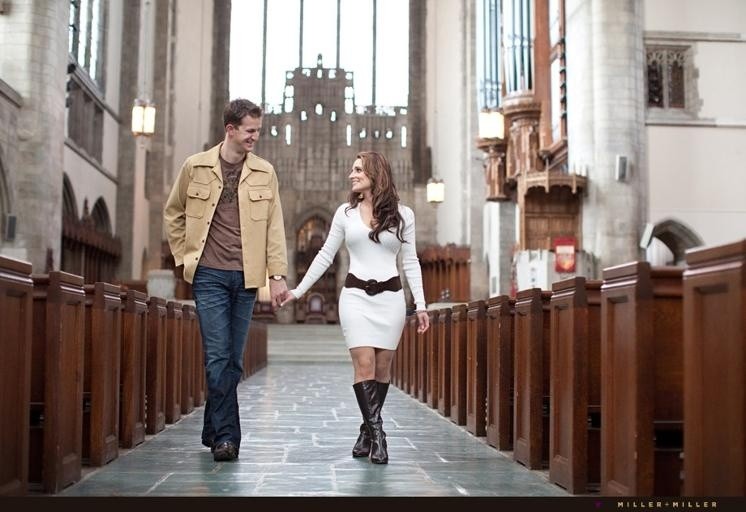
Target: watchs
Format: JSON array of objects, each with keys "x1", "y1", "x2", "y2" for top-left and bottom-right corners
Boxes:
[{"x1": 269, "y1": 275, "x2": 283, "y2": 281}]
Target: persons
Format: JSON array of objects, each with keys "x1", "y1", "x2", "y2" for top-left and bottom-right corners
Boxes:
[
  {"x1": 163, "y1": 98, "x2": 290, "y2": 463},
  {"x1": 278, "y1": 149, "x2": 432, "y2": 465}
]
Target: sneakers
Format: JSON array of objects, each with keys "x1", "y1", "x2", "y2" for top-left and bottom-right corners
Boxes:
[{"x1": 213, "y1": 441, "x2": 238, "y2": 461}]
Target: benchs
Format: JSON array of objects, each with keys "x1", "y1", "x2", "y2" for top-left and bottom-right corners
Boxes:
[
  {"x1": 1, "y1": 257, "x2": 267, "y2": 495},
  {"x1": 390, "y1": 237, "x2": 744, "y2": 498}
]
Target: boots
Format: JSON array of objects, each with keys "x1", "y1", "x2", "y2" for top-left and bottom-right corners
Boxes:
[{"x1": 352, "y1": 379, "x2": 389, "y2": 464}]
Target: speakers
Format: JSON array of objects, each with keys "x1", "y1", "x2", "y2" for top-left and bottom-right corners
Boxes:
[
  {"x1": 616, "y1": 155, "x2": 629, "y2": 180},
  {"x1": 5, "y1": 214, "x2": 16, "y2": 240}
]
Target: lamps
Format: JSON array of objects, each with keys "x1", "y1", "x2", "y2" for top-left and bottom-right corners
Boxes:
[{"x1": 132, "y1": 99, "x2": 157, "y2": 136}]
